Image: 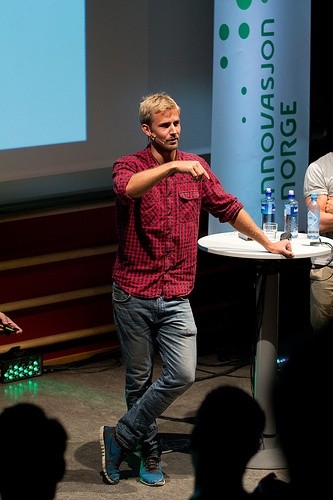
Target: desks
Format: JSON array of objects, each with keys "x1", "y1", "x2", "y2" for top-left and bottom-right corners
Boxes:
[{"x1": 197, "y1": 230, "x2": 333, "y2": 470}]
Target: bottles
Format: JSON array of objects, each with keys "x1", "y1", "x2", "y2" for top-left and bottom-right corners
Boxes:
[
  {"x1": 284, "y1": 190, "x2": 299, "y2": 239},
  {"x1": 261, "y1": 188, "x2": 276, "y2": 229},
  {"x1": 307, "y1": 193, "x2": 319, "y2": 239}
]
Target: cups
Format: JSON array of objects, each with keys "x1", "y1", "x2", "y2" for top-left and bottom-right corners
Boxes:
[{"x1": 263, "y1": 222, "x2": 278, "y2": 241}]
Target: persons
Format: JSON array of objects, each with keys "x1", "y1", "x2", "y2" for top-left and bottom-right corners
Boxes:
[
  {"x1": 0, "y1": 405, "x2": 68, "y2": 500},
  {"x1": 99, "y1": 92, "x2": 296, "y2": 486},
  {"x1": 187, "y1": 386, "x2": 267, "y2": 500},
  {"x1": 303, "y1": 151, "x2": 333, "y2": 332},
  {"x1": 0, "y1": 312, "x2": 24, "y2": 335}
]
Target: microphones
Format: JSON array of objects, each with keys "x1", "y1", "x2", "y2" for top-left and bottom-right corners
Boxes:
[{"x1": 150, "y1": 133, "x2": 165, "y2": 145}]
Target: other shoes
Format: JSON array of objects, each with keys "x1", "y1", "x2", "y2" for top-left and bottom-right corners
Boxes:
[
  {"x1": 99, "y1": 425, "x2": 125, "y2": 485},
  {"x1": 138, "y1": 458, "x2": 165, "y2": 486}
]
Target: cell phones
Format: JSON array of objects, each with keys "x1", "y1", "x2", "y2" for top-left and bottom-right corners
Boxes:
[{"x1": 0, "y1": 323, "x2": 15, "y2": 331}]
